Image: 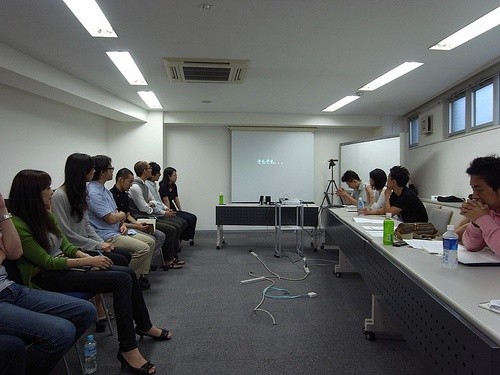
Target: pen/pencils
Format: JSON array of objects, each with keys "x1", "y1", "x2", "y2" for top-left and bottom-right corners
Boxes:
[{"x1": 97, "y1": 250, "x2": 104, "y2": 256}]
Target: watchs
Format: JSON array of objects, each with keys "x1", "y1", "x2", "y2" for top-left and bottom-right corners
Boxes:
[{"x1": 0, "y1": 213, "x2": 12, "y2": 221}]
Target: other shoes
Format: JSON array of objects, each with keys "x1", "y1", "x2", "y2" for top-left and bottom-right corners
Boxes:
[
  {"x1": 168, "y1": 258, "x2": 184, "y2": 269},
  {"x1": 138, "y1": 277, "x2": 150, "y2": 290},
  {"x1": 99, "y1": 309, "x2": 113, "y2": 320}
]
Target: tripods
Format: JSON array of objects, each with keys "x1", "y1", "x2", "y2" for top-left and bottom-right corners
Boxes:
[{"x1": 318, "y1": 160, "x2": 344, "y2": 214}]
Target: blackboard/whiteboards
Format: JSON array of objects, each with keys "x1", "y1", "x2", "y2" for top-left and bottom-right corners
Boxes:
[{"x1": 339, "y1": 133, "x2": 410, "y2": 194}]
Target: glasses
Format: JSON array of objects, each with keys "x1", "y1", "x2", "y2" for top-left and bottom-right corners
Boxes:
[{"x1": 106, "y1": 167, "x2": 114, "y2": 170}]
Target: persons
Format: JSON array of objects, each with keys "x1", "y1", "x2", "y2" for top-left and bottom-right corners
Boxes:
[
  {"x1": 357, "y1": 169, "x2": 387, "y2": 215},
  {"x1": 7, "y1": 169, "x2": 172, "y2": 375},
  {"x1": 460, "y1": 154, "x2": 500, "y2": 257},
  {"x1": 0, "y1": 191, "x2": 97, "y2": 375},
  {"x1": 335, "y1": 171, "x2": 367, "y2": 206},
  {"x1": 384, "y1": 166, "x2": 429, "y2": 223},
  {"x1": 0, "y1": 335, "x2": 25, "y2": 375},
  {"x1": 454, "y1": 217, "x2": 471, "y2": 242},
  {"x1": 109, "y1": 160, "x2": 198, "y2": 272},
  {"x1": 86, "y1": 155, "x2": 155, "y2": 290},
  {"x1": 51, "y1": 154, "x2": 131, "y2": 266}
]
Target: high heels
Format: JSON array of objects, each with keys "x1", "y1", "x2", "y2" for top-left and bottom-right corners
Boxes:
[
  {"x1": 135, "y1": 326, "x2": 171, "y2": 341},
  {"x1": 117, "y1": 350, "x2": 157, "y2": 375}
]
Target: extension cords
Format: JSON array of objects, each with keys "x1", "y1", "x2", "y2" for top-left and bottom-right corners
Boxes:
[
  {"x1": 248, "y1": 250, "x2": 259, "y2": 257},
  {"x1": 240, "y1": 277, "x2": 265, "y2": 285},
  {"x1": 303, "y1": 265, "x2": 310, "y2": 274}
]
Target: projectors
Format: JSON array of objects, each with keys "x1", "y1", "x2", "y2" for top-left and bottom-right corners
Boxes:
[{"x1": 279, "y1": 198, "x2": 300, "y2": 205}]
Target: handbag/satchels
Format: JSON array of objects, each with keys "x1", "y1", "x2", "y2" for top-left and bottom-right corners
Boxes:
[
  {"x1": 437, "y1": 195, "x2": 465, "y2": 202},
  {"x1": 396, "y1": 222, "x2": 438, "y2": 239}
]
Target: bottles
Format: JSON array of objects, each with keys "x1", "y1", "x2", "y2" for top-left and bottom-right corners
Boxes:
[
  {"x1": 84, "y1": 335, "x2": 97, "y2": 374},
  {"x1": 357, "y1": 198, "x2": 364, "y2": 217},
  {"x1": 383, "y1": 213, "x2": 394, "y2": 245},
  {"x1": 442, "y1": 225, "x2": 458, "y2": 268},
  {"x1": 218, "y1": 192, "x2": 223, "y2": 205}
]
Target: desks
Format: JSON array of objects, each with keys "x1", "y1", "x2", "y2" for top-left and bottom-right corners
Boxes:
[
  {"x1": 216, "y1": 203, "x2": 319, "y2": 258},
  {"x1": 321, "y1": 203, "x2": 500, "y2": 347}
]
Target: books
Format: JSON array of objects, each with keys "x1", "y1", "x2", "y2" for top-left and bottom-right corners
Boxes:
[{"x1": 456, "y1": 243, "x2": 500, "y2": 267}]
